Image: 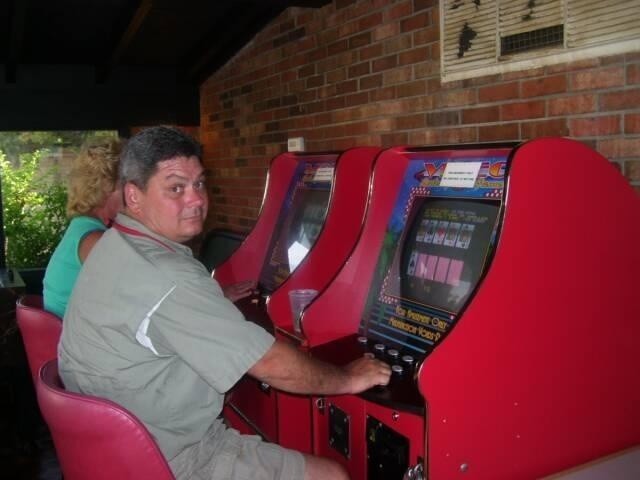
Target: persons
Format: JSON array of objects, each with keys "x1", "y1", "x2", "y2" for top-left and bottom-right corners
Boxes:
[
  {"x1": 41, "y1": 134, "x2": 128, "y2": 321},
  {"x1": 56, "y1": 125, "x2": 393, "y2": 480}
]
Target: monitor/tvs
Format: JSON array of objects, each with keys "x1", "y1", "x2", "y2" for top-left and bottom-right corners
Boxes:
[
  {"x1": 383, "y1": 196, "x2": 502, "y2": 317},
  {"x1": 257, "y1": 189, "x2": 332, "y2": 292}
]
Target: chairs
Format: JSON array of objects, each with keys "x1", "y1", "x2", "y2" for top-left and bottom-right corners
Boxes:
[
  {"x1": 33, "y1": 357, "x2": 176, "y2": 480},
  {"x1": 13, "y1": 293, "x2": 64, "y2": 389}
]
